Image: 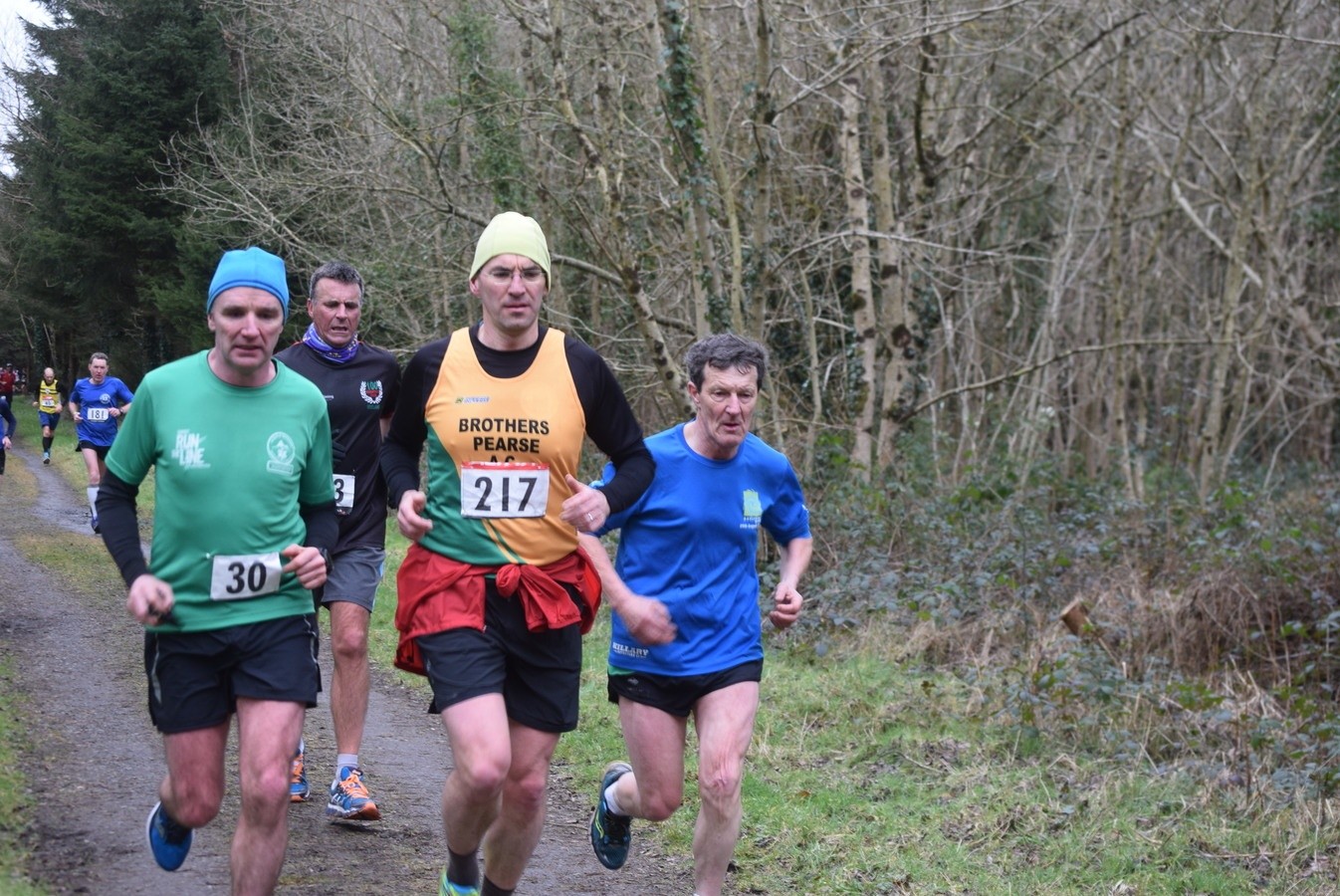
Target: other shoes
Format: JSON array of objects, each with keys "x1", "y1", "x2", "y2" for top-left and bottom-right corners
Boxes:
[
  {"x1": 42, "y1": 453, "x2": 50, "y2": 465},
  {"x1": 439, "y1": 867, "x2": 479, "y2": 896},
  {"x1": 91, "y1": 518, "x2": 100, "y2": 533}
]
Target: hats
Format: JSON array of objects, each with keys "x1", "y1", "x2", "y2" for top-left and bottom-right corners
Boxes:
[
  {"x1": 468, "y1": 211, "x2": 552, "y2": 294},
  {"x1": 206, "y1": 246, "x2": 289, "y2": 331}
]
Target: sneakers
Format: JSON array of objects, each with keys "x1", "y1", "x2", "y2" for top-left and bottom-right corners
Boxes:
[
  {"x1": 588, "y1": 760, "x2": 633, "y2": 870},
  {"x1": 288, "y1": 740, "x2": 311, "y2": 802},
  {"x1": 144, "y1": 800, "x2": 192, "y2": 872},
  {"x1": 326, "y1": 767, "x2": 381, "y2": 821}
]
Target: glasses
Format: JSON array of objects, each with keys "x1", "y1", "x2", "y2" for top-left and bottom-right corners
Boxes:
[{"x1": 479, "y1": 269, "x2": 545, "y2": 286}]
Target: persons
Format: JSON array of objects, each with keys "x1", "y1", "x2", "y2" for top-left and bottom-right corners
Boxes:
[
  {"x1": 70, "y1": 353, "x2": 133, "y2": 533},
  {"x1": 96, "y1": 247, "x2": 344, "y2": 896},
  {"x1": 0, "y1": 362, "x2": 28, "y2": 476},
  {"x1": 576, "y1": 335, "x2": 814, "y2": 896},
  {"x1": 32, "y1": 367, "x2": 69, "y2": 464},
  {"x1": 388, "y1": 210, "x2": 659, "y2": 896},
  {"x1": 268, "y1": 260, "x2": 401, "y2": 820}
]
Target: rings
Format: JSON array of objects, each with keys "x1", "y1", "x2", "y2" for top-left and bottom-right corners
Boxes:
[{"x1": 587, "y1": 512, "x2": 593, "y2": 521}]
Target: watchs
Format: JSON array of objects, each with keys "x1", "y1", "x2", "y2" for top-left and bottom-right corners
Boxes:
[{"x1": 118, "y1": 407, "x2": 124, "y2": 416}]
